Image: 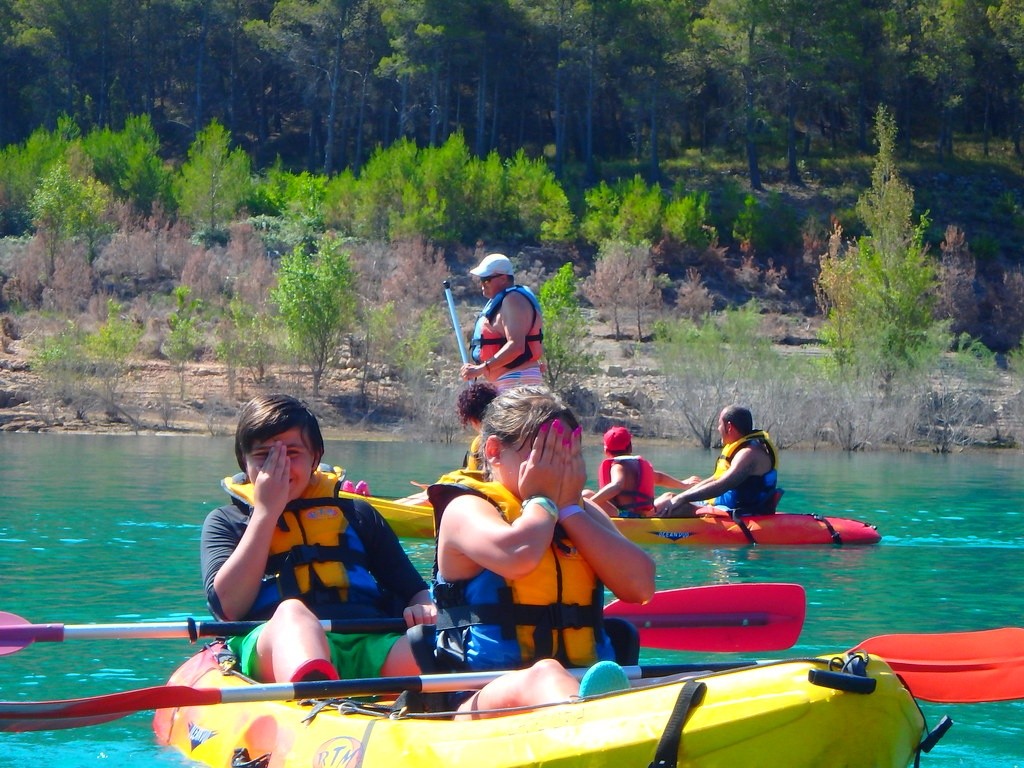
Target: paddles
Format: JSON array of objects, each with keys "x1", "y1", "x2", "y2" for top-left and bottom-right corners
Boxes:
[
  {"x1": 442, "y1": 280, "x2": 473, "y2": 385},
  {"x1": 1, "y1": 625, "x2": 1024, "y2": 737},
  {"x1": 1, "y1": 581, "x2": 808, "y2": 657},
  {"x1": 695, "y1": 499, "x2": 729, "y2": 516}
]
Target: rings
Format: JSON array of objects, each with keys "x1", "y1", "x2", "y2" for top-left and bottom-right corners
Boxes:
[{"x1": 665, "y1": 508, "x2": 668, "y2": 511}]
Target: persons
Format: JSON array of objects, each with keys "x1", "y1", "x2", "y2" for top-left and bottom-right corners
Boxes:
[
  {"x1": 343, "y1": 253, "x2": 778, "y2": 518},
  {"x1": 200, "y1": 386, "x2": 656, "y2": 721}
]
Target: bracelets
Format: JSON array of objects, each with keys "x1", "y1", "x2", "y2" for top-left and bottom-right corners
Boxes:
[
  {"x1": 520, "y1": 495, "x2": 553, "y2": 514},
  {"x1": 558, "y1": 504, "x2": 585, "y2": 523},
  {"x1": 484, "y1": 360, "x2": 490, "y2": 373}
]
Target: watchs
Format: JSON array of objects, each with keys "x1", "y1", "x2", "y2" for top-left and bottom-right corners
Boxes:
[{"x1": 528, "y1": 495, "x2": 558, "y2": 522}]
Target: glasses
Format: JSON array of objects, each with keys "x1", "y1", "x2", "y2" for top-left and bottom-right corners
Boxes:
[{"x1": 479, "y1": 274, "x2": 502, "y2": 282}]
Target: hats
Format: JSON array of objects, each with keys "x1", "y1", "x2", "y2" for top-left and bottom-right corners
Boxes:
[
  {"x1": 604, "y1": 426, "x2": 633, "y2": 451},
  {"x1": 469, "y1": 253, "x2": 513, "y2": 277}
]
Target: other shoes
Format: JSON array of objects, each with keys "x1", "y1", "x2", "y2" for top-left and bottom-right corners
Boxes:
[
  {"x1": 390, "y1": 688, "x2": 450, "y2": 719},
  {"x1": 287, "y1": 657, "x2": 340, "y2": 682},
  {"x1": 579, "y1": 661, "x2": 631, "y2": 699}
]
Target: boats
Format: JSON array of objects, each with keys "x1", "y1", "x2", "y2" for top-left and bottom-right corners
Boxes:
[
  {"x1": 152, "y1": 639, "x2": 953, "y2": 768},
  {"x1": 332, "y1": 480, "x2": 883, "y2": 545}
]
